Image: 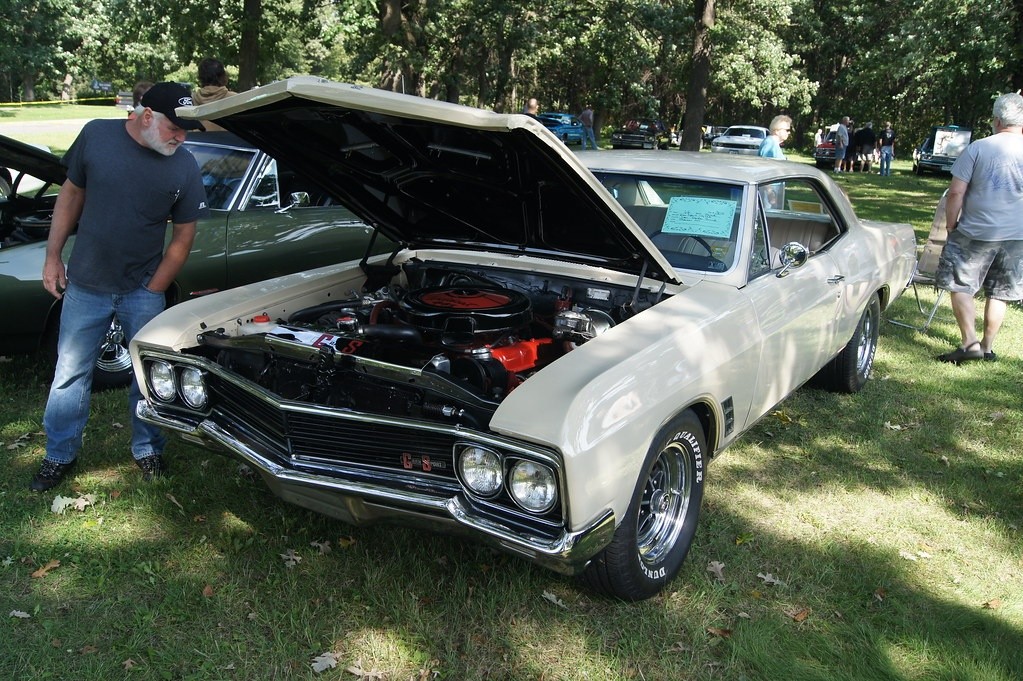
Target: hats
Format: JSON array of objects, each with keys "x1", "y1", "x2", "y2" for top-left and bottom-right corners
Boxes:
[{"x1": 141, "y1": 80, "x2": 206, "y2": 133}]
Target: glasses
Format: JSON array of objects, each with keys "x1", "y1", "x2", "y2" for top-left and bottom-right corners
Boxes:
[
  {"x1": 773, "y1": 128, "x2": 791, "y2": 132},
  {"x1": 990, "y1": 114, "x2": 995, "y2": 122}
]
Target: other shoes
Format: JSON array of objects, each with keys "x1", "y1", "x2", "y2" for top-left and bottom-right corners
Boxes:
[
  {"x1": 834, "y1": 170, "x2": 844, "y2": 173},
  {"x1": 944, "y1": 341, "x2": 984, "y2": 364},
  {"x1": 984, "y1": 350, "x2": 996, "y2": 361}
]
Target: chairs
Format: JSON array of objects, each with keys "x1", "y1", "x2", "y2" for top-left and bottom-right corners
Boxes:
[{"x1": 879, "y1": 188, "x2": 964, "y2": 333}]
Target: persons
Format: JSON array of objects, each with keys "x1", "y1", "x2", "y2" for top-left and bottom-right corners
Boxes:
[
  {"x1": 524, "y1": 98, "x2": 564, "y2": 143},
  {"x1": 577, "y1": 106, "x2": 597, "y2": 150},
  {"x1": 758, "y1": 115, "x2": 792, "y2": 211},
  {"x1": 188, "y1": 58, "x2": 239, "y2": 132},
  {"x1": 934, "y1": 93, "x2": 1023, "y2": 364},
  {"x1": 30, "y1": 81, "x2": 209, "y2": 494},
  {"x1": 814, "y1": 116, "x2": 896, "y2": 176},
  {"x1": 133, "y1": 81, "x2": 155, "y2": 109}
]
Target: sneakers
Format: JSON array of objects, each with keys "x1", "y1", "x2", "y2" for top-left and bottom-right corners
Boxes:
[
  {"x1": 30, "y1": 454, "x2": 78, "y2": 494},
  {"x1": 135, "y1": 454, "x2": 163, "y2": 482}
]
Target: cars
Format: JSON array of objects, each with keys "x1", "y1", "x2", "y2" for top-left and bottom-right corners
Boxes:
[
  {"x1": 612, "y1": 120, "x2": 673, "y2": 151},
  {"x1": 536, "y1": 113, "x2": 588, "y2": 147},
  {"x1": 0, "y1": 73, "x2": 916, "y2": 605},
  {"x1": 710, "y1": 125, "x2": 786, "y2": 158},
  {"x1": 912, "y1": 125, "x2": 977, "y2": 176},
  {"x1": 814, "y1": 132, "x2": 869, "y2": 170}
]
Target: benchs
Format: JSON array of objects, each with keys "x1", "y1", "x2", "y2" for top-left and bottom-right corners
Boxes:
[{"x1": 623, "y1": 206, "x2": 831, "y2": 274}]
